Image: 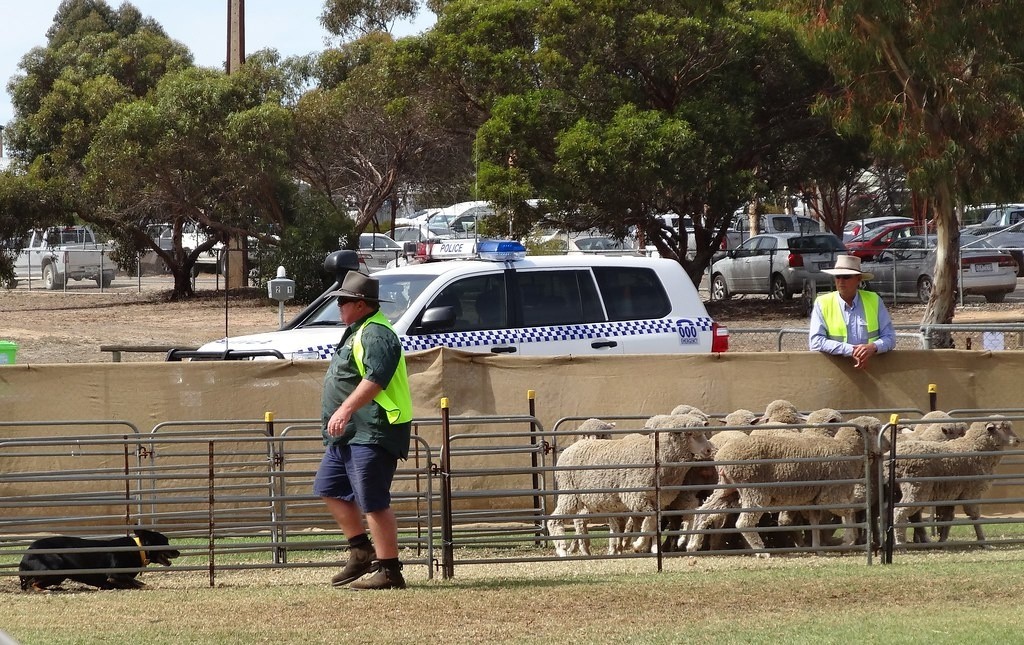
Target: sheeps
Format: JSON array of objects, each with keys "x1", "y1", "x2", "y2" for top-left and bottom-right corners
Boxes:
[{"x1": 546, "y1": 398, "x2": 1021, "y2": 559}]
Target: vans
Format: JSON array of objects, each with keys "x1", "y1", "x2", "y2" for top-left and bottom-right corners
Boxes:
[
  {"x1": 630, "y1": 214, "x2": 704, "y2": 244},
  {"x1": 429, "y1": 199, "x2": 548, "y2": 239},
  {"x1": 928, "y1": 203, "x2": 1024, "y2": 229},
  {"x1": 520, "y1": 212, "x2": 632, "y2": 247}
]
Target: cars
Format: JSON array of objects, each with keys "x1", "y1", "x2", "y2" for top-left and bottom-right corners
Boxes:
[
  {"x1": 857, "y1": 234, "x2": 1017, "y2": 303},
  {"x1": 844, "y1": 222, "x2": 935, "y2": 263},
  {"x1": 843, "y1": 216, "x2": 915, "y2": 244},
  {"x1": 384, "y1": 226, "x2": 460, "y2": 261},
  {"x1": 567, "y1": 235, "x2": 646, "y2": 258},
  {"x1": 395, "y1": 208, "x2": 445, "y2": 226},
  {"x1": 708, "y1": 229, "x2": 853, "y2": 300},
  {"x1": 356, "y1": 232, "x2": 403, "y2": 276}
]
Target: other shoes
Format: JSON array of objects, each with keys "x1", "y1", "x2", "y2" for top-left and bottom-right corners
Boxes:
[{"x1": 330, "y1": 537, "x2": 406, "y2": 591}]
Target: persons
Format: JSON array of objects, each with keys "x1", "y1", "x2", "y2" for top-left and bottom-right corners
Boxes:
[
  {"x1": 808, "y1": 254, "x2": 897, "y2": 369},
  {"x1": 311, "y1": 268, "x2": 414, "y2": 590}
]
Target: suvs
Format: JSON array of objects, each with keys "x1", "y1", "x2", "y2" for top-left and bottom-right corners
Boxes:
[
  {"x1": 171, "y1": 221, "x2": 281, "y2": 278},
  {"x1": 164, "y1": 215, "x2": 729, "y2": 362}
]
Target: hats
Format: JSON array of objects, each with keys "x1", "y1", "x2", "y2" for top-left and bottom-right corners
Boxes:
[
  {"x1": 819, "y1": 255, "x2": 875, "y2": 280},
  {"x1": 323, "y1": 270, "x2": 397, "y2": 303}
]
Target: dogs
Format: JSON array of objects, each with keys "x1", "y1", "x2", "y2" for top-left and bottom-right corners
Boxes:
[{"x1": 19, "y1": 529, "x2": 180, "y2": 594}]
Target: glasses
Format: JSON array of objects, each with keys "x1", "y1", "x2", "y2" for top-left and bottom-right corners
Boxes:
[{"x1": 833, "y1": 274, "x2": 862, "y2": 279}]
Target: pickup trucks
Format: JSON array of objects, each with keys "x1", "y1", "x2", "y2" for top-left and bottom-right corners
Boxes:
[
  {"x1": 126, "y1": 223, "x2": 173, "y2": 277},
  {"x1": 1, "y1": 225, "x2": 118, "y2": 290},
  {"x1": 686, "y1": 214, "x2": 827, "y2": 257}
]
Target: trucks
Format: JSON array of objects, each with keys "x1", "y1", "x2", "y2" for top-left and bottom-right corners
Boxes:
[{"x1": 959, "y1": 208, "x2": 1024, "y2": 277}]
